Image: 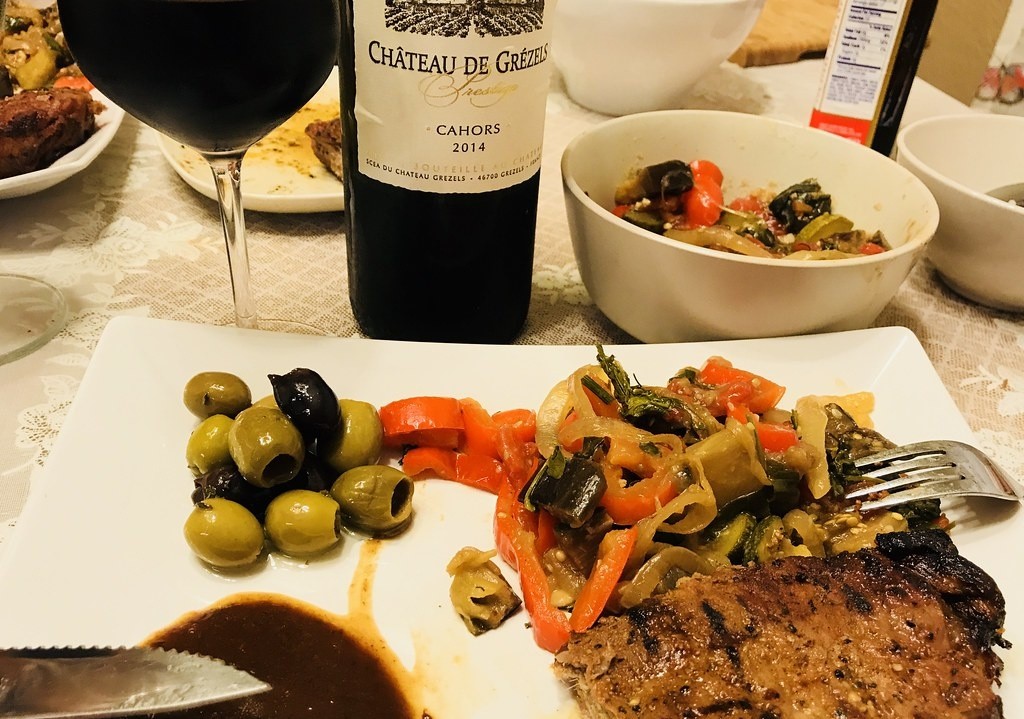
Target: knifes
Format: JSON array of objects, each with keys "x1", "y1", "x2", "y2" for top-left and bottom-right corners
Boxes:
[{"x1": 0, "y1": 643, "x2": 275, "y2": 719}]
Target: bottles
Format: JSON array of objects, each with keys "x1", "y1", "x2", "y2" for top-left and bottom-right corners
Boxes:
[
  {"x1": 339, "y1": 0, "x2": 556, "y2": 346},
  {"x1": 809, "y1": 0, "x2": 942, "y2": 158}
]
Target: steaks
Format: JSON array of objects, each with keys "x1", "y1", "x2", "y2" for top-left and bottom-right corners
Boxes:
[
  {"x1": 553, "y1": 528, "x2": 1005, "y2": 719},
  {"x1": 305, "y1": 117, "x2": 344, "y2": 183},
  {"x1": 0, "y1": 87, "x2": 105, "y2": 180}
]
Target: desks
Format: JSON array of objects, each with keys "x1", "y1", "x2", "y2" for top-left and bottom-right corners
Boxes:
[{"x1": 1, "y1": 51, "x2": 1023, "y2": 718}]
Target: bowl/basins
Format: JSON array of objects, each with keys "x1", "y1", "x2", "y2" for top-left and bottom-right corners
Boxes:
[
  {"x1": 560, "y1": 107, "x2": 941, "y2": 344},
  {"x1": 545, "y1": 0, "x2": 768, "y2": 117},
  {"x1": 891, "y1": 112, "x2": 1024, "y2": 315}
]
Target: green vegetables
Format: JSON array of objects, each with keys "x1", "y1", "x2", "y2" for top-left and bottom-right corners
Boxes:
[
  {"x1": 582, "y1": 342, "x2": 942, "y2": 517},
  {"x1": 739, "y1": 178, "x2": 834, "y2": 250},
  {"x1": 5, "y1": 15, "x2": 75, "y2": 68}
]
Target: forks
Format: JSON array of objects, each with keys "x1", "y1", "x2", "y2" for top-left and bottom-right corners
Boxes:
[{"x1": 843, "y1": 439, "x2": 1023, "y2": 515}]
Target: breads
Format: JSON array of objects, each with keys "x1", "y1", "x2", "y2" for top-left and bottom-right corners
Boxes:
[{"x1": 722, "y1": 1, "x2": 843, "y2": 66}]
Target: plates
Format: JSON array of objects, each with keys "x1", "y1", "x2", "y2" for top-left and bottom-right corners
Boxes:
[
  {"x1": 0, "y1": 309, "x2": 1024, "y2": 719},
  {"x1": 154, "y1": 61, "x2": 347, "y2": 215},
  {"x1": 0, "y1": 67, "x2": 127, "y2": 197}
]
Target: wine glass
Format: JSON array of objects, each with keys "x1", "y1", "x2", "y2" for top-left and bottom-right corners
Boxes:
[{"x1": 56, "y1": 0, "x2": 344, "y2": 338}]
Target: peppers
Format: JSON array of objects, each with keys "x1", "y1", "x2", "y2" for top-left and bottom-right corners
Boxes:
[
  {"x1": 685, "y1": 160, "x2": 723, "y2": 228},
  {"x1": 380, "y1": 356, "x2": 798, "y2": 653}
]
